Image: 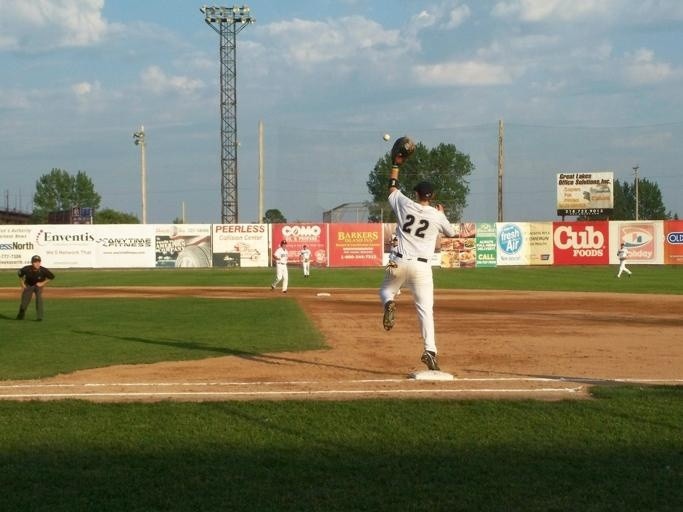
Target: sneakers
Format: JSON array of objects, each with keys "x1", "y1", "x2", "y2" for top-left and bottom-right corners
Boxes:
[
  {"x1": 305, "y1": 275, "x2": 309, "y2": 277},
  {"x1": 272, "y1": 286, "x2": 286, "y2": 293},
  {"x1": 383, "y1": 300, "x2": 396, "y2": 330},
  {"x1": 421, "y1": 351, "x2": 439, "y2": 370}
]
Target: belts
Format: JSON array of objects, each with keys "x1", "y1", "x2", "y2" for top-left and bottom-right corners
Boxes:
[{"x1": 396, "y1": 253, "x2": 427, "y2": 262}]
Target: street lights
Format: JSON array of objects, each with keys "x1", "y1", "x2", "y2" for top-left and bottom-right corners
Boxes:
[
  {"x1": 633, "y1": 164, "x2": 641, "y2": 220},
  {"x1": 132, "y1": 124, "x2": 147, "y2": 223},
  {"x1": 201, "y1": 5, "x2": 256, "y2": 222}
]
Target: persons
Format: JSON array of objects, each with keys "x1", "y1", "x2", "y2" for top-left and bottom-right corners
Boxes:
[
  {"x1": 271, "y1": 240, "x2": 290, "y2": 294},
  {"x1": 298, "y1": 245, "x2": 313, "y2": 278},
  {"x1": 15, "y1": 256, "x2": 55, "y2": 321},
  {"x1": 616, "y1": 243, "x2": 632, "y2": 279},
  {"x1": 381, "y1": 135, "x2": 458, "y2": 373},
  {"x1": 386, "y1": 236, "x2": 402, "y2": 296}
]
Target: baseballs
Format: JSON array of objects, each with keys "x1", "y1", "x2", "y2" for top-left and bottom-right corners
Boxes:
[{"x1": 384, "y1": 133, "x2": 391, "y2": 142}]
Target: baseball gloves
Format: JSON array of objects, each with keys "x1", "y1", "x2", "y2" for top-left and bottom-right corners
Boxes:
[{"x1": 390, "y1": 135, "x2": 417, "y2": 167}]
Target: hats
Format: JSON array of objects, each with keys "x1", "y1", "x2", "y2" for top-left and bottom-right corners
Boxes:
[
  {"x1": 281, "y1": 240, "x2": 287, "y2": 244},
  {"x1": 32, "y1": 255, "x2": 41, "y2": 262},
  {"x1": 303, "y1": 246, "x2": 307, "y2": 247},
  {"x1": 621, "y1": 243, "x2": 625, "y2": 246},
  {"x1": 413, "y1": 182, "x2": 433, "y2": 199}
]
